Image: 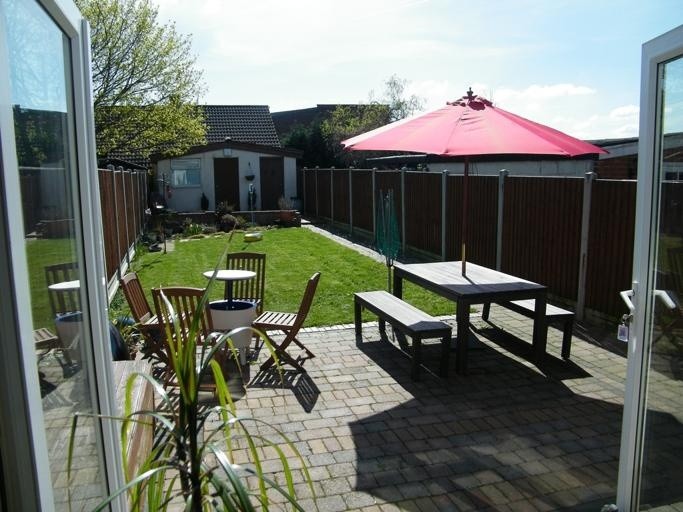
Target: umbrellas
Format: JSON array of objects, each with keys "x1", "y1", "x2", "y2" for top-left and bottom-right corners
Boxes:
[{"x1": 340, "y1": 87, "x2": 610, "y2": 276}]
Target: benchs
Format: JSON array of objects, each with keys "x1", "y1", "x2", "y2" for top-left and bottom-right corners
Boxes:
[
  {"x1": 482, "y1": 298, "x2": 576, "y2": 358},
  {"x1": 353, "y1": 290, "x2": 453, "y2": 383}
]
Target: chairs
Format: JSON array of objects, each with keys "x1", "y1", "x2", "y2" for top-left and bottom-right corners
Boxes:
[
  {"x1": 34, "y1": 328, "x2": 60, "y2": 379},
  {"x1": 653, "y1": 270, "x2": 683, "y2": 361},
  {"x1": 120, "y1": 252, "x2": 321, "y2": 406},
  {"x1": 44, "y1": 263, "x2": 79, "y2": 365},
  {"x1": 667, "y1": 248, "x2": 683, "y2": 296}
]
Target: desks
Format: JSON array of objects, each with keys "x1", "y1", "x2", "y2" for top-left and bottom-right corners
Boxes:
[
  {"x1": 392, "y1": 260, "x2": 549, "y2": 376},
  {"x1": 48, "y1": 279, "x2": 88, "y2": 380}
]
